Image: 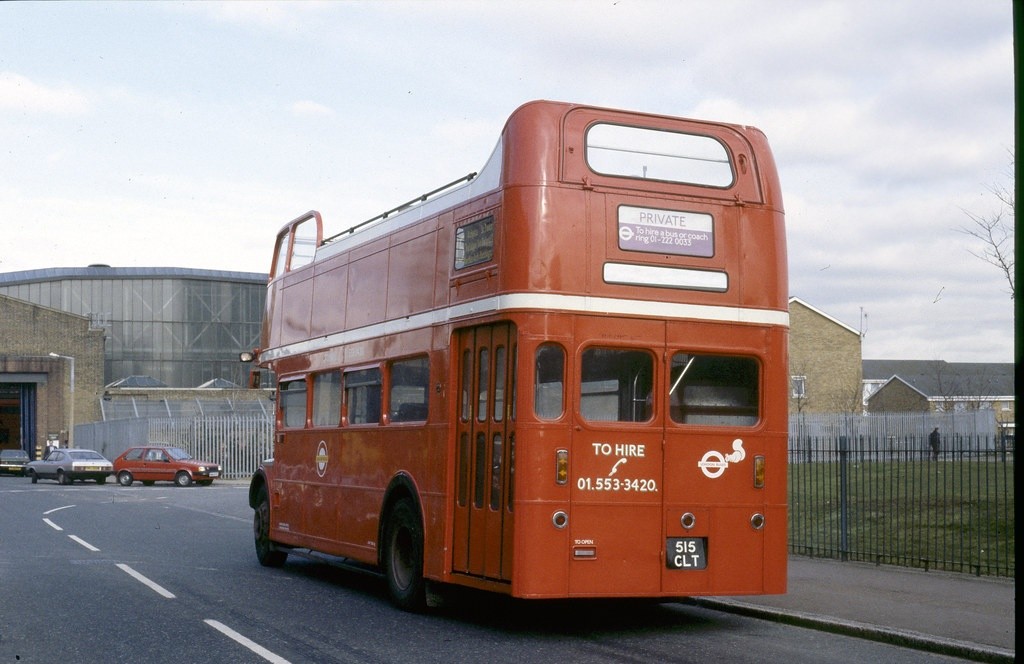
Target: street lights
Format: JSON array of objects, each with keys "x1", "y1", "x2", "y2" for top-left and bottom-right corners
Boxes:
[{"x1": 50, "y1": 352, "x2": 75, "y2": 448}]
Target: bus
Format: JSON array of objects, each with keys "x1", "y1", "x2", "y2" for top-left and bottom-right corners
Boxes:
[{"x1": 248, "y1": 99, "x2": 790, "y2": 620}]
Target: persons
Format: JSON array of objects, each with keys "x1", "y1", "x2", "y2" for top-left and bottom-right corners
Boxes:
[
  {"x1": 44, "y1": 440, "x2": 58, "y2": 455},
  {"x1": 929, "y1": 427, "x2": 940, "y2": 463}
]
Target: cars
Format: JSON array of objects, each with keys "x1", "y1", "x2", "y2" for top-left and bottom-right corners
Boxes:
[
  {"x1": 113, "y1": 446, "x2": 224, "y2": 488},
  {"x1": 24, "y1": 448, "x2": 113, "y2": 485},
  {"x1": 0, "y1": 449, "x2": 32, "y2": 477}
]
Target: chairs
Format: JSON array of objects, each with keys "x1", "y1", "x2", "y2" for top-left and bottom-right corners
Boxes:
[
  {"x1": 397, "y1": 403, "x2": 428, "y2": 422},
  {"x1": 151, "y1": 452, "x2": 157, "y2": 461}
]
